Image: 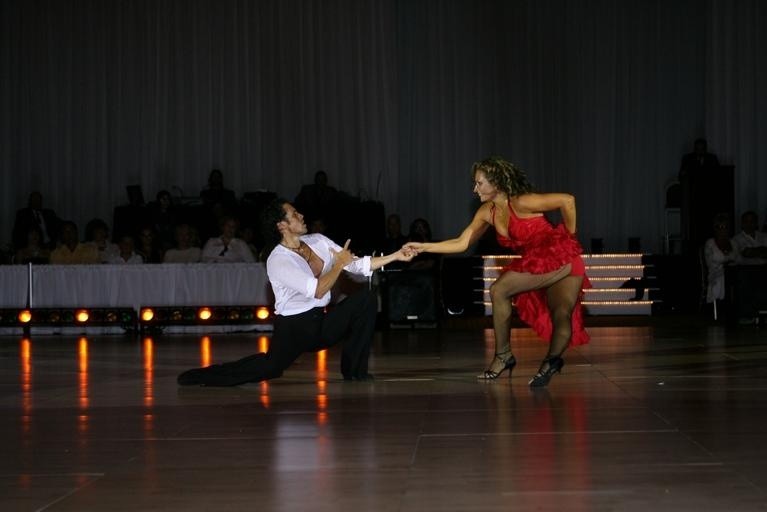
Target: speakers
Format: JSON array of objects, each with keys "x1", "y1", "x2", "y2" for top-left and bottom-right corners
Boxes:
[{"x1": 378, "y1": 239, "x2": 447, "y2": 330}]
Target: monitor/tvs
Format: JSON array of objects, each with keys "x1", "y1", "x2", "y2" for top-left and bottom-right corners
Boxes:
[{"x1": 126, "y1": 184, "x2": 145, "y2": 207}]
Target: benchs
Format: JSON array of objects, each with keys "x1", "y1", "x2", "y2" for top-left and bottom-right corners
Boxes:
[
  {"x1": 0, "y1": 263, "x2": 277, "y2": 333},
  {"x1": 477, "y1": 252, "x2": 659, "y2": 315}
]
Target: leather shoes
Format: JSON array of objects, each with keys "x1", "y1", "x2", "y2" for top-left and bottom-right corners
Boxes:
[
  {"x1": 342, "y1": 368, "x2": 373, "y2": 383},
  {"x1": 177, "y1": 368, "x2": 205, "y2": 387}
]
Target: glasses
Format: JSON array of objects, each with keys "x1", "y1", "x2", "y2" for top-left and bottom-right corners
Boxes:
[{"x1": 217, "y1": 246, "x2": 229, "y2": 256}]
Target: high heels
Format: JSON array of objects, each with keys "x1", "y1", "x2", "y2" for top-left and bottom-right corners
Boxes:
[
  {"x1": 528, "y1": 356, "x2": 565, "y2": 386},
  {"x1": 479, "y1": 351, "x2": 517, "y2": 379}
]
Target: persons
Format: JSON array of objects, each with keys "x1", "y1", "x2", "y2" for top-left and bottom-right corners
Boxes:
[
  {"x1": 13, "y1": 170, "x2": 257, "y2": 264},
  {"x1": 403, "y1": 157, "x2": 590, "y2": 384},
  {"x1": 696, "y1": 211, "x2": 766, "y2": 324},
  {"x1": 296, "y1": 171, "x2": 445, "y2": 328},
  {"x1": 177, "y1": 198, "x2": 416, "y2": 384}
]
